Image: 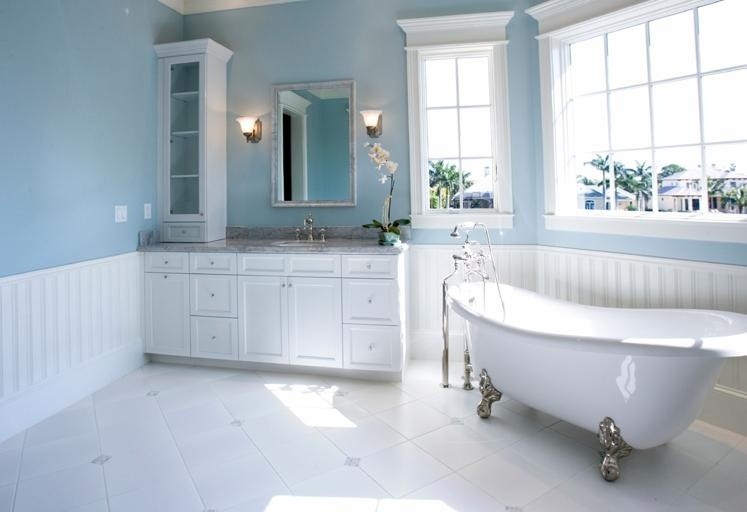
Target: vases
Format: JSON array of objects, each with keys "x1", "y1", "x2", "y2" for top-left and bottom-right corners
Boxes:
[{"x1": 378, "y1": 232, "x2": 402, "y2": 246}]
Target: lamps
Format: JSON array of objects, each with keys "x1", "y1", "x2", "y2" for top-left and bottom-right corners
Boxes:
[
  {"x1": 361, "y1": 110, "x2": 382, "y2": 139},
  {"x1": 236, "y1": 117, "x2": 262, "y2": 143}
]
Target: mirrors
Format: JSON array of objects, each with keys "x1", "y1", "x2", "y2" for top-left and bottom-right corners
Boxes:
[{"x1": 270, "y1": 80, "x2": 356, "y2": 207}]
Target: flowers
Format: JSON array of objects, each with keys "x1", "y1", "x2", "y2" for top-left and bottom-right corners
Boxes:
[{"x1": 362, "y1": 142, "x2": 412, "y2": 235}]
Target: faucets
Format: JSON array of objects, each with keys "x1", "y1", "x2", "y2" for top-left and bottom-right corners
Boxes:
[
  {"x1": 472, "y1": 266, "x2": 489, "y2": 283},
  {"x1": 306, "y1": 216, "x2": 313, "y2": 240}
]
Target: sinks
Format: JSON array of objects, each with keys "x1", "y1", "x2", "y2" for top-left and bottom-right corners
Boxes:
[{"x1": 282, "y1": 241, "x2": 325, "y2": 245}]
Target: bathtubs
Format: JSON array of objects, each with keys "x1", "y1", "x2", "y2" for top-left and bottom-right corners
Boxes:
[{"x1": 447, "y1": 277, "x2": 747, "y2": 482}]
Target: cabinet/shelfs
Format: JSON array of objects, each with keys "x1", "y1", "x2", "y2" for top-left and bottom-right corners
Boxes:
[
  {"x1": 154, "y1": 38, "x2": 234, "y2": 243},
  {"x1": 238, "y1": 253, "x2": 342, "y2": 379},
  {"x1": 143, "y1": 252, "x2": 190, "y2": 366},
  {"x1": 191, "y1": 252, "x2": 239, "y2": 370},
  {"x1": 344, "y1": 253, "x2": 411, "y2": 382}
]
